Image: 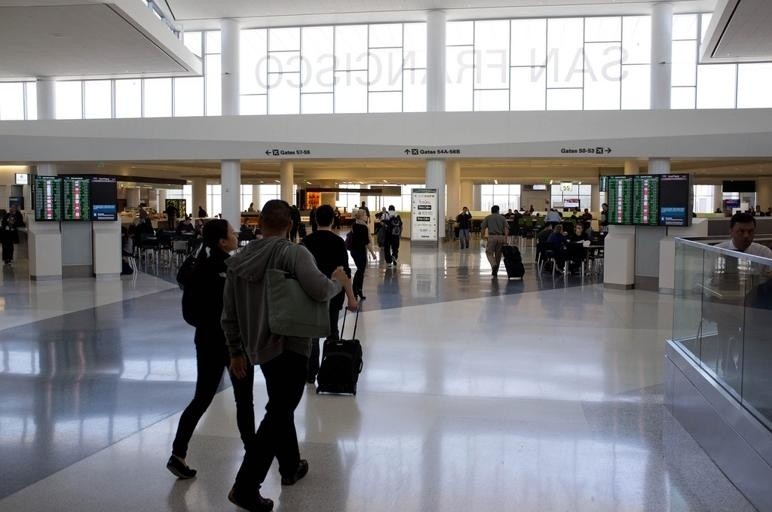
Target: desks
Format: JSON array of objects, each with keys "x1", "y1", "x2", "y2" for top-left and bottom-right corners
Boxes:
[{"x1": 698, "y1": 274, "x2": 768, "y2": 377}]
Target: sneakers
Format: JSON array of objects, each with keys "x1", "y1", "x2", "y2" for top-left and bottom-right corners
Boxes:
[{"x1": 167, "y1": 456, "x2": 196, "y2": 478}]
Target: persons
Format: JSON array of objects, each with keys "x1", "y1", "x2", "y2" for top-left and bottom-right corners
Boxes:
[
  {"x1": 123, "y1": 202, "x2": 218, "y2": 252},
  {"x1": 0, "y1": 201, "x2": 26, "y2": 267},
  {"x1": 457, "y1": 207, "x2": 472, "y2": 249},
  {"x1": 222, "y1": 198, "x2": 349, "y2": 511},
  {"x1": 705, "y1": 205, "x2": 771, "y2": 301},
  {"x1": 286, "y1": 204, "x2": 319, "y2": 242},
  {"x1": 165, "y1": 217, "x2": 264, "y2": 480},
  {"x1": 294, "y1": 203, "x2": 359, "y2": 385},
  {"x1": 239, "y1": 202, "x2": 262, "y2": 241},
  {"x1": 481, "y1": 204, "x2": 607, "y2": 277},
  {"x1": 333, "y1": 201, "x2": 403, "y2": 301}
]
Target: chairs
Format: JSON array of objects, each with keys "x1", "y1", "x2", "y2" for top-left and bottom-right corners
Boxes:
[
  {"x1": 126, "y1": 217, "x2": 260, "y2": 271},
  {"x1": 455, "y1": 216, "x2": 603, "y2": 278},
  {"x1": 696, "y1": 243, "x2": 721, "y2": 375}
]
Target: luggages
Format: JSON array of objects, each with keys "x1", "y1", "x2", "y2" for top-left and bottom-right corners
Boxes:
[
  {"x1": 317, "y1": 307, "x2": 361, "y2": 395},
  {"x1": 502, "y1": 246, "x2": 525, "y2": 279}
]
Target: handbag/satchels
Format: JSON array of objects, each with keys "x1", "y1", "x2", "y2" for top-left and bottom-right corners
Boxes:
[
  {"x1": 266, "y1": 270, "x2": 332, "y2": 337},
  {"x1": 344, "y1": 231, "x2": 353, "y2": 250},
  {"x1": 177, "y1": 254, "x2": 210, "y2": 326}
]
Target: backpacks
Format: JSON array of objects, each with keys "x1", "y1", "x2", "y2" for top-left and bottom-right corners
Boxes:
[{"x1": 390, "y1": 214, "x2": 400, "y2": 235}]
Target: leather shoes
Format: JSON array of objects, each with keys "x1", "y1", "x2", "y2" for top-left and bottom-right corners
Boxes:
[
  {"x1": 281, "y1": 460, "x2": 308, "y2": 484},
  {"x1": 228, "y1": 487, "x2": 273, "y2": 511}
]
